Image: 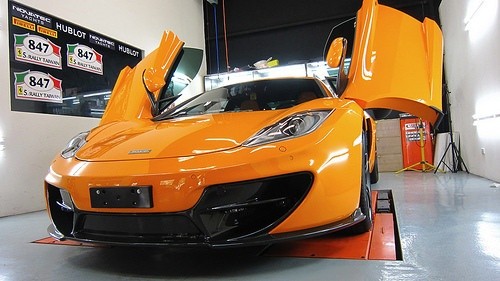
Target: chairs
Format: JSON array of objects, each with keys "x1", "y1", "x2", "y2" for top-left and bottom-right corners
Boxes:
[
  {"x1": 296, "y1": 91, "x2": 316, "y2": 105},
  {"x1": 241, "y1": 99, "x2": 259, "y2": 112}
]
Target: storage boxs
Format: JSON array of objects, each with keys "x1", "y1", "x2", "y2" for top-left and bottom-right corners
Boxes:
[{"x1": 253, "y1": 60, "x2": 279, "y2": 70}]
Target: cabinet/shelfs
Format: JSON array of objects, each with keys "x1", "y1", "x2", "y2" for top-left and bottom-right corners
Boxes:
[{"x1": 373, "y1": 119, "x2": 404, "y2": 174}]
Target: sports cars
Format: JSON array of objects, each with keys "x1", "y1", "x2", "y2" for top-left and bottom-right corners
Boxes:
[{"x1": 43, "y1": 1, "x2": 444, "y2": 250}]
